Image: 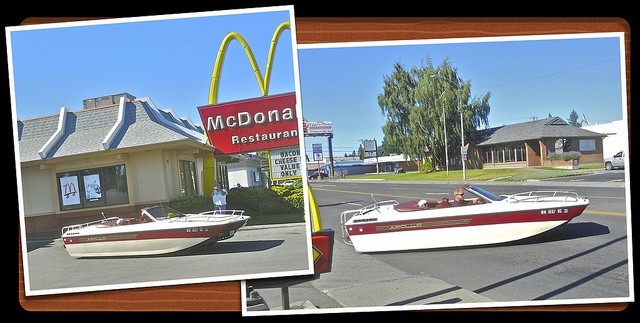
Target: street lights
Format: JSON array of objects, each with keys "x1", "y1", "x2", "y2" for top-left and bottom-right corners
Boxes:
[
  {"x1": 431, "y1": 74, "x2": 467, "y2": 181},
  {"x1": 433, "y1": 94, "x2": 450, "y2": 175}
]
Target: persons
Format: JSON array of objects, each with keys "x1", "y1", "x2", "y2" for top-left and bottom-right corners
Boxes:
[
  {"x1": 418, "y1": 199, "x2": 429, "y2": 208},
  {"x1": 451, "y1": 189, "x2": 482, "y2": 205},
  {"x1": 141, "y1": 211, "x2": 150, "y2": 222},
  {"x1": 237, "y1": 182, "x2": 241, "y2": 188},
  {"x1": 211, "y1": 181, "x2": 228, "y2": 213}
]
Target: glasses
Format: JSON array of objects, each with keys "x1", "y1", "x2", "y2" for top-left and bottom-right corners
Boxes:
[{"x1": 457, "y1": 193, "x2": 464, "y2": 196}]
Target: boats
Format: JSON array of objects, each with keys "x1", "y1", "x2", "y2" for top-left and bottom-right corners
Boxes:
[
  {"x1": 341, "y1": 184, "x2": 590, "y2": 252},
  {"x1": 61, "y1": 205, "x2": 251, "y2": 259}
]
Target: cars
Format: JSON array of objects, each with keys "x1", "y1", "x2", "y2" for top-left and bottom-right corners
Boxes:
[
  {"x1": 604, "y1": 151, "x2": 625, "y2": 170},
  {"x1": 279, "y1": 180, "x2": 295, "y2": 184},
  {"x1": 309, "y1": 172, "x2": 328, "y2": 179}
]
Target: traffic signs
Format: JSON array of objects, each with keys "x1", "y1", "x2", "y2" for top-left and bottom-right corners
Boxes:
[
  {"x1": 364, "y1": 139, "x2": 377, "y2": 157},
  {"x1": 313, "y1": 143, "x2": 323, "y2": 160}
]
